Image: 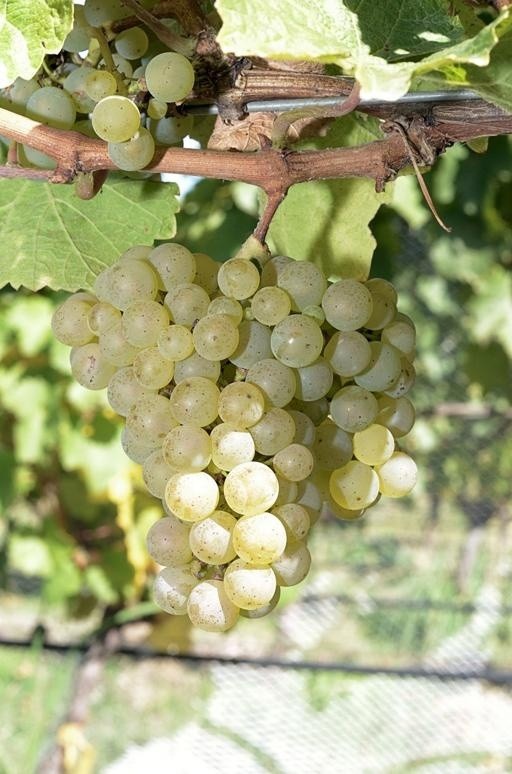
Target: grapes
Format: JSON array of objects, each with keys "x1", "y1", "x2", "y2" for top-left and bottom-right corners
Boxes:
[
  {"x1": 5, "y1": 0, "x2": 201, "y2": 179},
  {"x1": 51, "y1": 240, "x2": 418, "y2": 634}
]
